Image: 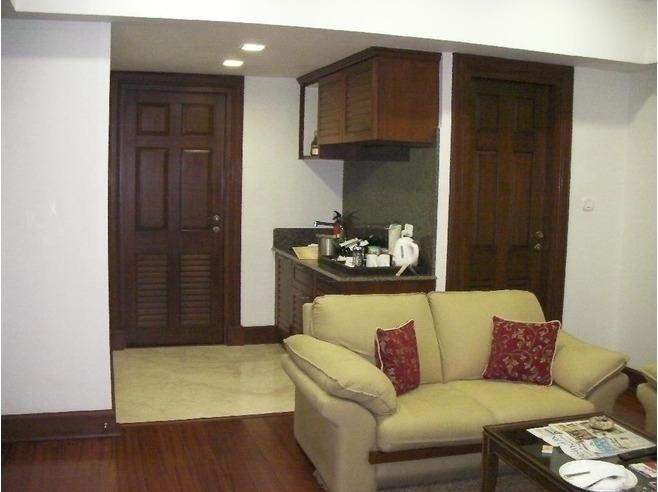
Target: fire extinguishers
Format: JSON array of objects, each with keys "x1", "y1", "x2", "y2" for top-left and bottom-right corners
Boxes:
[{"x1": 333, "y1": 211, "x2": 343, "y2": 234}]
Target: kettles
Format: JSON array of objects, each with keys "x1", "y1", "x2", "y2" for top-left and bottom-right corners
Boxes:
[{"x1": 392, "y1": 235, "x2": 420, "y2": 267}]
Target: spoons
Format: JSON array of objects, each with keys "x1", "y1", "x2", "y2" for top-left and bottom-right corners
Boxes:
[{"x1": 580, "y1": 475, "x2": 618, "y2": 489}]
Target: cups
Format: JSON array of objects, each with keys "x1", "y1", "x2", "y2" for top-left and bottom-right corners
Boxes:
[{"x1": 366, "y1": 254, "x2": 391, "y2": 267}]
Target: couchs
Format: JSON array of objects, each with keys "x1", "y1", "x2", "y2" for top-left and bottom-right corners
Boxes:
[{"x1": 281, "y1": 288, "x2": 630, "y2": 492}]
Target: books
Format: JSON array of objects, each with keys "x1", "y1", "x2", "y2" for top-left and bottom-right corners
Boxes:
[{"x1": 629, "y1": 461, "x2": 657, "y2": 479}]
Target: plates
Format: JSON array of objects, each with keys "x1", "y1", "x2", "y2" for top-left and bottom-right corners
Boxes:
[{"x1": 559, "y1": 459, "x2": 638, "y2": 492}]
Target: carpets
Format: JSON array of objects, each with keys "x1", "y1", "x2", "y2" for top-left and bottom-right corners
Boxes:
[{"x1": 112, "y1": 341, "x2": 295, "y2": 423}]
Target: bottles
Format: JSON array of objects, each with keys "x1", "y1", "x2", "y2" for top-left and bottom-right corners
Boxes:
[
  {"x1": 311, "y1": 128, "x2": 318, "y2": 156},
  {"x1": 337, "y1": 230, "x2": 378, "y2": 256},
  {"x1": 353, "y1": 246, "x2": 363, "y2": 267}
]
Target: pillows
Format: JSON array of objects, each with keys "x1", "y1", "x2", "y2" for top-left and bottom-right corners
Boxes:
[
  {"x1": 482, "y1": 315, "x2": 561, "y2": 386},
  {"x1": 374, "y1": 319, "x2": 420, "y2": 397}
]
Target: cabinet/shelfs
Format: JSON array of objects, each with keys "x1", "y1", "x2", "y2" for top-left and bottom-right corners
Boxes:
[
  {"x1": 316, "y1": 57, "x2": 440, "y2": 148},
  {"x1": 275, "y1": 251, "x2": 438, "y2": 343}
]
